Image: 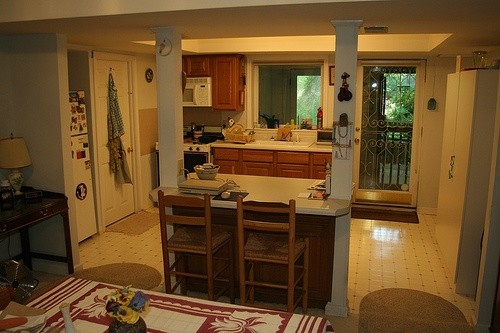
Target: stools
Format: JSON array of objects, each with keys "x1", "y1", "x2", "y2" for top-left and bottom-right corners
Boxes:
[
  {"x1": 157, "y1": 189, "x2": 237, "y2": 304},
  {"x1": 236, "y1": 194, "x2": 310, "y2": 314}
]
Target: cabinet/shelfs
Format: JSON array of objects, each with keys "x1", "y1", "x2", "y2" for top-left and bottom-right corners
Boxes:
[
  {"x1": 0, "y1": 186, "x2": 74, "y2": 274},
  {"x1": 182, "y1": 54, "x2": 247, "y2": 113},
  {"x1": 211, "y1": 147, "x2": 332, "y2": 179}
]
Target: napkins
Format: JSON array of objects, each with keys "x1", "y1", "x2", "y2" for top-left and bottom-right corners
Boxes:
[{"x1": 3, "y1": 314, "x2": 45, "y2": 332}]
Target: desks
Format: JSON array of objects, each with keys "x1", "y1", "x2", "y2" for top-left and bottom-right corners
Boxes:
[
  {"x1": 149, "y1": 172, "x2": 355, "y2": 318},
  {"x1": 11, "y1": 273, "x2": 335, "y2": 333}
]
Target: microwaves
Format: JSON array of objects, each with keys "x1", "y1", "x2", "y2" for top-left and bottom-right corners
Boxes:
[{"x1": 182, "y1": 77, "x2": 212, "y2": 107}]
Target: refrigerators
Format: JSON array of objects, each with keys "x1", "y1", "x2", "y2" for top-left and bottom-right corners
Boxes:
[{"x1": 67, "y1": 90, "x2": 98, "y2": 243}]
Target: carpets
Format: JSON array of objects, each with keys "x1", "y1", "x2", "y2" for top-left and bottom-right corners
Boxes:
[
  {"x1": 106, "y1": 209, "x2": 160, "y2": 236},
  {"x1": 359, "y1": 288, "x2": 475, "y2": 333},
  {"x1": 73, "y1": 262, "x2": 162, "y2": 290},
  {"x1": 350, "y1": 207, "x2": 420, "y2": 224}
]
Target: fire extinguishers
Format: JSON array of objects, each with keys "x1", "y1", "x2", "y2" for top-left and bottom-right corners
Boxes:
[{"x1": 317, "y1": 107, "x2": 323, "y2": 130}]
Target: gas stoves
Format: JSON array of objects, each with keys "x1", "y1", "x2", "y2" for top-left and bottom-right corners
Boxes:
[{"x1": 183, "y1": 125, "x2": 223, "y2": 153}]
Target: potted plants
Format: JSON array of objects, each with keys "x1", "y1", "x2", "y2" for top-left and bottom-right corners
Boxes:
[{"x1": 259, "y1": 113, "x2": 280, "y2": 129}]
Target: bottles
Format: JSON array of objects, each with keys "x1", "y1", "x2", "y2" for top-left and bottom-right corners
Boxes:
[
  {"x1": 291, "y1": 119, "x2": 294, "y2": 128},
  {"x1": 473, "y1": 51, "x2": 486, "y2": 67}
]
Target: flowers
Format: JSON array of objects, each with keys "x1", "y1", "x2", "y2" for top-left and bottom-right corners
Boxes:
[{"x1": 104, "y1": 285, "x2": 150, "y2": 324}]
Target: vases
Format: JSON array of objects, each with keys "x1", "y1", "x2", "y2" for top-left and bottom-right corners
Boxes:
[{"x1": 108, "y1": 316, "x2": 147, "y2": 333}]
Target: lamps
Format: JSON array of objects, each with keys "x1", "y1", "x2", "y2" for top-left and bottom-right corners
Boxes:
[{"x1": 0, "y1": 133, "x2": 32, "y2": 195}]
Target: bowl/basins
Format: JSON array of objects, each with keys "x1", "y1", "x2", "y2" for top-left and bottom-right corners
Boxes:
[{"x1": 194, "y1": 164, "x2": 220, "y2": 179}]
